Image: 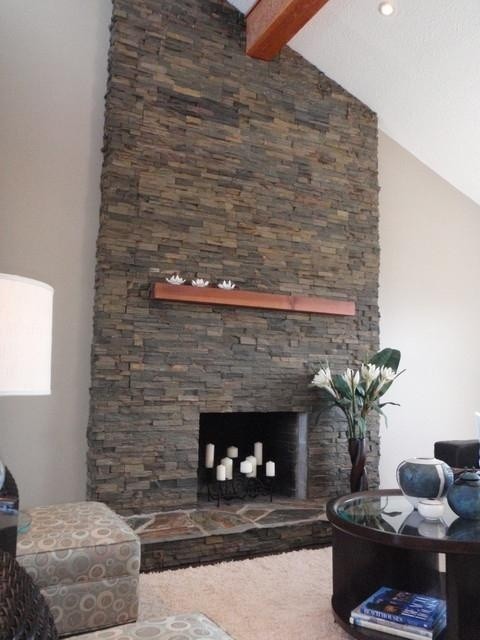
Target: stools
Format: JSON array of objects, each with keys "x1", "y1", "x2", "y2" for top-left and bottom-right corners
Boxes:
[{"x1": 434, "y1": 438, "x2": 480, "y2": 471}]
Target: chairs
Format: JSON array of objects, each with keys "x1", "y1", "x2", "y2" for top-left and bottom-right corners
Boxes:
[{"x1": 0, "y1": 500, "x2": 237, "y2": 640}]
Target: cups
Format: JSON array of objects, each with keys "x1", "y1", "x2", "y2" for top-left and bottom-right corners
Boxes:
[{"x1": 416, "y1": 498, "x2": 446, "y2": 521}]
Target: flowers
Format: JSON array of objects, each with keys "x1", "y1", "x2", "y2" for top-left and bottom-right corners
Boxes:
[{"x1": 302, "y1": 348, "x2": 406, "y2": 438}]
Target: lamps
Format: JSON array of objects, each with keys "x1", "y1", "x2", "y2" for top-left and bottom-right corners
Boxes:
[{"x1": 0, "y1": 270, "x2": 58, "y2": 491}]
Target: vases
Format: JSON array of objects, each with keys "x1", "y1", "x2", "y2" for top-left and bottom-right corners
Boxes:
[{"x1": 348, "y1": 438, "x2": 369, "y2": 493}]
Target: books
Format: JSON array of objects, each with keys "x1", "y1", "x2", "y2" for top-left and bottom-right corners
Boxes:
[
  {"x1": 351, "y1": 599, "x2": 447, "y2": 638},
  {"x1": 361, "y1": 584, "x2": 448, "y2": 630},
  {"x1": 349, "y1": 617, "x2": 448, "y2": 640}
]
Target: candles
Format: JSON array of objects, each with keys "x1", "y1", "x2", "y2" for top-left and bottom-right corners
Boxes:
[{"x1": 205, "y1": 441, "x2": 276, "y2": 482}]
